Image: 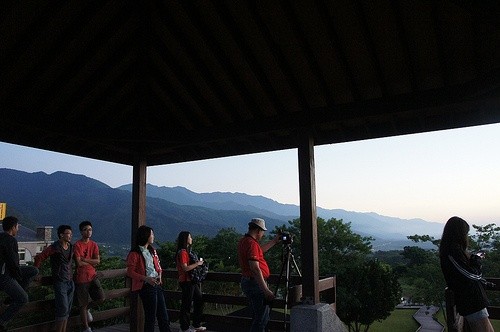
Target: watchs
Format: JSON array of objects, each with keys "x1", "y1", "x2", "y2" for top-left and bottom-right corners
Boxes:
[{"x1": 144, "y1": 277, "x2": 148, "y2": 284}]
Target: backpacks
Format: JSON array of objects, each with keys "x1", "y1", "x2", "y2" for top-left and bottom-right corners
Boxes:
[{"x1": 177, "y1": 247, "x2": 208, "y2": 281}]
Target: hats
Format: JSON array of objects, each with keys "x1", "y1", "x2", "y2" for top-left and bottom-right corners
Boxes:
[{"x1": 248, "y1": 218, "x2": 267, "y2": 231}]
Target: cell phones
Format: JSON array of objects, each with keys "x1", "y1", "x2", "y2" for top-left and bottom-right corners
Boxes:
[{"x1": 476, "y1": 251, "x2": 484, "y2": 257}]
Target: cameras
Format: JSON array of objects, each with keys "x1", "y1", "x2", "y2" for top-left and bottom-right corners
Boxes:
[{"x1": 279, "y1": 233, "x2": 294, "y2": 246}]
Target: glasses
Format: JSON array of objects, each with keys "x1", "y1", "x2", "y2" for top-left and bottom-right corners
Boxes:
[{"x1": 82, "y1": 228, "x2": 92, "y2": 231}]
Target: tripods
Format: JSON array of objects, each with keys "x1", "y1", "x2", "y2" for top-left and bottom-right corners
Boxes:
[{"x1": 268, "y1": 246, "x2": 302, "y2": 332}]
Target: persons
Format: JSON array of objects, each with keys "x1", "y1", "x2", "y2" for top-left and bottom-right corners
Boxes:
[
  {"x1": 72, "y1": 221, "x2": 105, "y2": 332},
  {"x1": 237, "y1": 217, "x2": 281, "y2": 332},
  {"x1": 0, "y1": 216, "x2": 39, "y2": 332},
  {"x1": 440, "y1": 217, "x2": 494, "y2": 332},
  {"x1": 34, "y1": 225, "x2": 74, "y2": 332},
  {"x1": 175, "y1": 230, "x2": 206, "y2": 332},
  {"x1": 126, "y1": 225, "x2": 172, "y2": 332}
]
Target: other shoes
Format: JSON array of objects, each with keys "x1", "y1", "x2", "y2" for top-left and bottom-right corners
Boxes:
[
  {"x1": 194, "y1": 326, "x2": 206, "y2": 330},
  {"x1": 87, "y1": 309, "x2": 93, "y2": 322},
  {"x1": 83, "y1": 327, "x2": 92, "y2": 332},
  {"x1": 179, "y1": 329, "x2": 196, "y2": 332}
]
